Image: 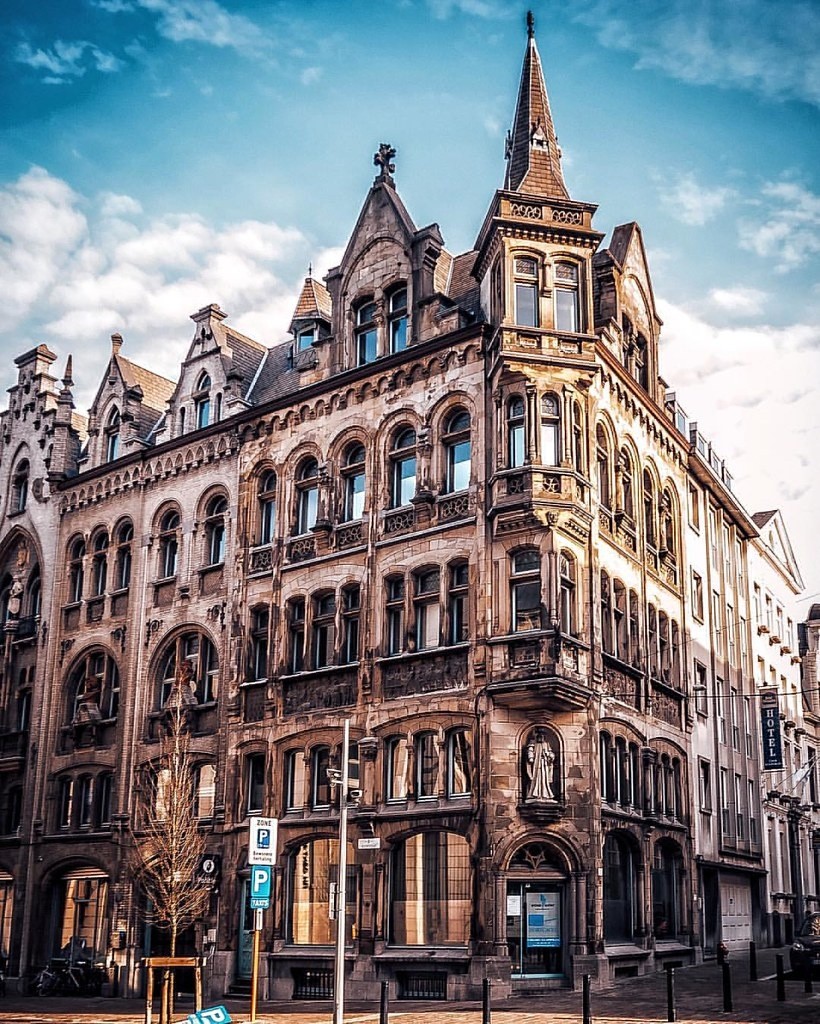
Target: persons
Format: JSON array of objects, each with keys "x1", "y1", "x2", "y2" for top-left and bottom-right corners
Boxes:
[{"x1": 529, "y1": 730, "x2": 556, "y2": 799}]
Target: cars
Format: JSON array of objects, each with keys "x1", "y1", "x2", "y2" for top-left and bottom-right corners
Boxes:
[{"x1": 790, "y1": 912, "x2": 820, "y2": 976}]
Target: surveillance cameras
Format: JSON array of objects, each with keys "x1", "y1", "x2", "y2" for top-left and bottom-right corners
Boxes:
[
  {"x1": 326, "y1": 768, "x2": 341, "y2": 778},
  {"x1": 351, "y1": 790, "x2": 363, "y2": 797}
]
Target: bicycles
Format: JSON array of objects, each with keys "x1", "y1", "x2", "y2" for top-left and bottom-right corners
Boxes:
[{"x1": 17, "y1": 964, "x2": 62, "y2": 998}]
